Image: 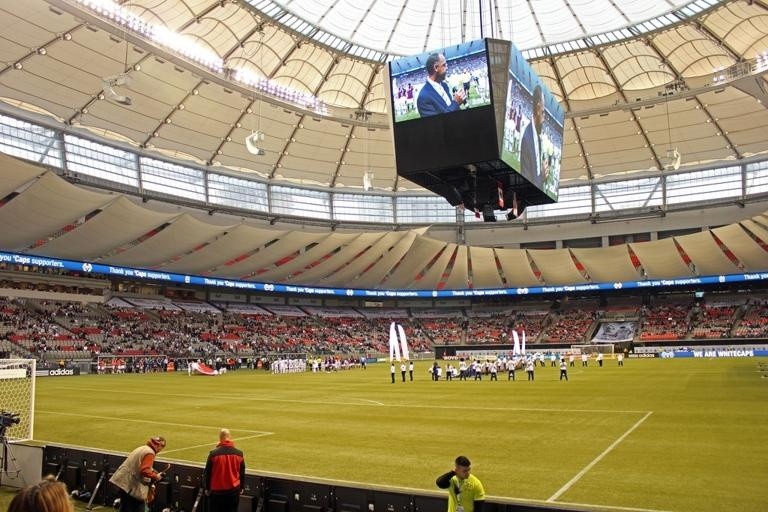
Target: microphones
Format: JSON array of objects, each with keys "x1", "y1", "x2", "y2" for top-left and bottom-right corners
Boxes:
[
  {"x1": 450, "y1": 81, "x2": 470, "y2": 109},
  {"x1": 542, "y1": 152, "x2": 549, "y2": 186}
]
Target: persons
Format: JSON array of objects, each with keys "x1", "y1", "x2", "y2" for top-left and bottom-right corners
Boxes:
[
  {"x1": 7, "y1": 474, "x2": 75, "y2": 512},
  {"x1": 391, "y1": 52, "x2": 490, "y2": 117},
  {"x1": 502, "y1": 80, "x2": 563, "y2": 194},
  {"x1": 107, "y1": 437, "x2": 166, "y2": 512},
  {"x1": 202, "y1": 428, "x2": 246, "y2": 512},
  {"x1": 436, "y1": 456, "x2": 485, "y2": 512},
  {"x1": 2, "y1": 263, "x2": 768, "y2": 384}
]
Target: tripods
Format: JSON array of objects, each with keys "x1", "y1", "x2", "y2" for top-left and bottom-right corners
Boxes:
[{"x1": 0, "y1": 438, "x2": 28, "y2": 487}]
